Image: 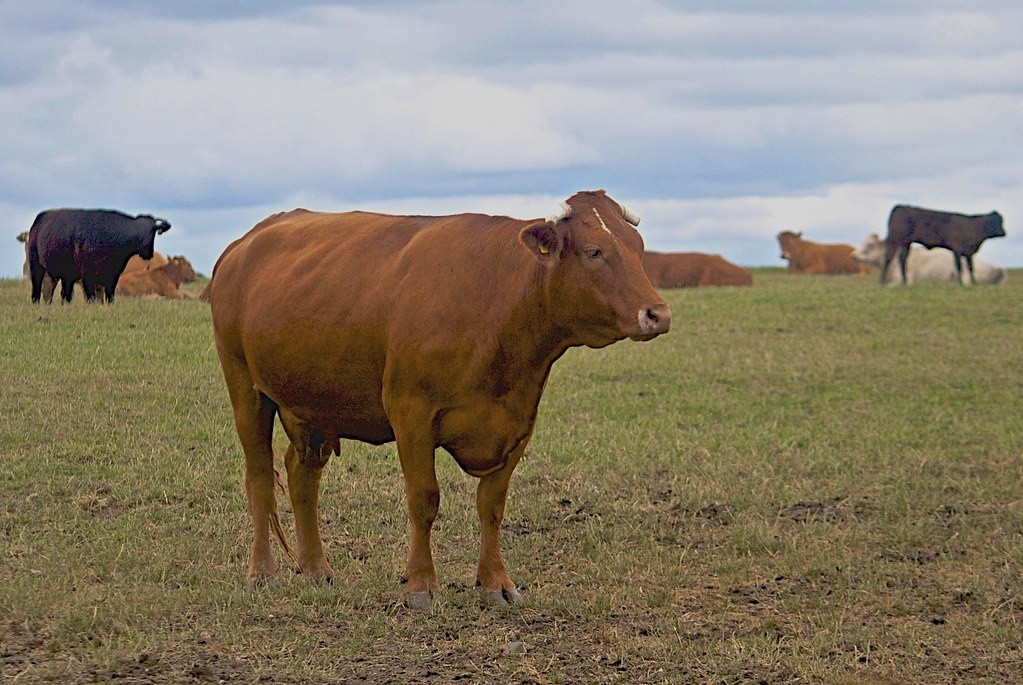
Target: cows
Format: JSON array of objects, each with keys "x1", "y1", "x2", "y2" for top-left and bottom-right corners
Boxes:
[
  {"x1": 875, "y1": 204, "x2": 1007, "y2": 288},
  {"x1": 16, "y1": 207, "x2": 197, "y2": 307},
  {"x1": 198, "y1": 188, "x2": 672, "y2": 612},
  {"x1": 641, "y1": 252, "x2": 752, "y2": 290},
  {"x1": 775, "y1": 230, "x2": 869, "y2": 275},
  {"x1": 848, "y1": 232, "x2": 1003, "y2": 286}
]
